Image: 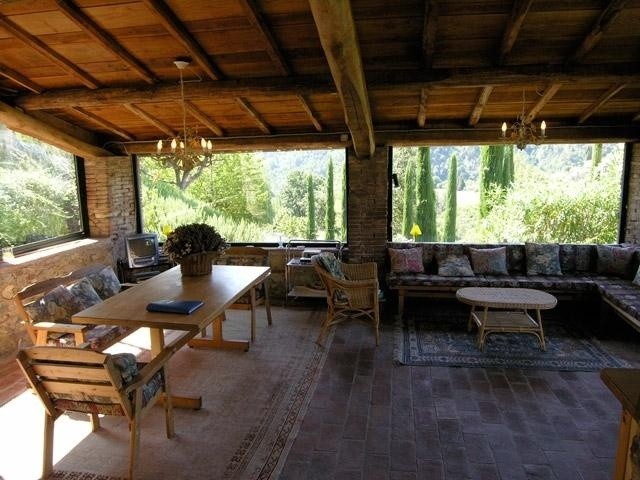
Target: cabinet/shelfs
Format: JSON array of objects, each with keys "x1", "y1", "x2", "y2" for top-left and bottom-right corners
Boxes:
[
  {"x1": 118, "y1": 257, "x2": 174, "y2": 284},
  {"x1": 600, "y1": 368, "x2": 640, "y2": 480}
]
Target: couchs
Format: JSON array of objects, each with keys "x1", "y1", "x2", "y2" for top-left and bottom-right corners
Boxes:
[{"x1": 385, "y1": 242, "x2": 640, "y2": 334}]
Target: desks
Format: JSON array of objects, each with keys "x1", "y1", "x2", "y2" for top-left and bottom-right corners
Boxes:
[{"x1": 71, "y1": 265, "x2": 270, "y2": 410}]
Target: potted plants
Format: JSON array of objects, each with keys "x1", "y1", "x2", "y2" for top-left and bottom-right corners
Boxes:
[{"x1": 161, "y1": 222, "x2": 230, "y2": 276}]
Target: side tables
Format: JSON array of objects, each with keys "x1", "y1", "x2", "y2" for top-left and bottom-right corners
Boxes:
[{"x1": 284, "y1": 258, "x2": 327, "y2": 306}]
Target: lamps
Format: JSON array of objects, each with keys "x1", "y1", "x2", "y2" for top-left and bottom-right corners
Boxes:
[
  {"x1": 150, "y1": 61, "x2": 214, "y2": 174},
  {"x1": 498, "y1": 88, "x2": 548, "y2": 151}
]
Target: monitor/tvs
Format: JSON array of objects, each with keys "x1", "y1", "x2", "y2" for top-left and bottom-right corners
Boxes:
[{"x1": 125, "y1": 234, "x2": 158, "y2": 268}]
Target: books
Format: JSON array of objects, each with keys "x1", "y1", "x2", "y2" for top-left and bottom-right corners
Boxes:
[{"x1": 147, "y1": 299, "x2": 204, "y2": 314}]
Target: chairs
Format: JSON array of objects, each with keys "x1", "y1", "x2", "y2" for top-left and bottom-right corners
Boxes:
[
  {"x1": 311, "y1": 255, "x2": 380, "y2": 346},
  {"x1": 16, "y1": 346, "x2": 175, "y2": 480},
  {"x1": 201, "y1": 247, "x2": 272, "y2": 343}
]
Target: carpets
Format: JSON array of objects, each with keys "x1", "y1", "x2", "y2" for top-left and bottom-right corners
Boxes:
[
  {"x1": 392, "y1": 313, "x2": 633, "y2": 372},
  {"x1": 0, "y1": 307, "x2": 335, "y2": 478}
]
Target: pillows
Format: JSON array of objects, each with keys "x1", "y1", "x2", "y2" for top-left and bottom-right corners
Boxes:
[
  {"x1": 597, "y1": 244, "x2": 635, "y2": 277},
  {"x1": 632, "y1": 264, "x2": 640, "y2": 287},
  {"x1": 23, "y1": 285, "x2": 86, "y2": 339},
  {"x1": 389, "y1": 247, "x2": 425, "y2": 272},
  {"x1": 67, "y1": 278, "x2": 103, "y2": 331},
  {"x1": 525, "y1": 242, "x2": 562, "y2": 276},
  {"x1": 216, "y1": 255, "x2": 265, "y2": 289},
  {"x1": 93, "y1": 265, "x2": 122, "y2": 300},
  {"x1": 469, "y1": 247, "x2": 508, "y2": 275},
  {"x1": 436, "y1": 253, "x2": 475, "y2": 277},
  {"x1": 319, "y1": 252, "x2": 344, "y2": 299},
  {"x1": 110, "y1": 354, "x2": 139, "y2": 384}
]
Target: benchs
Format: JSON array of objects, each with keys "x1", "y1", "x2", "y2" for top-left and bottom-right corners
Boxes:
[{"x1": 13, "y1": 264, "x2": 141, "y2": 353}]
